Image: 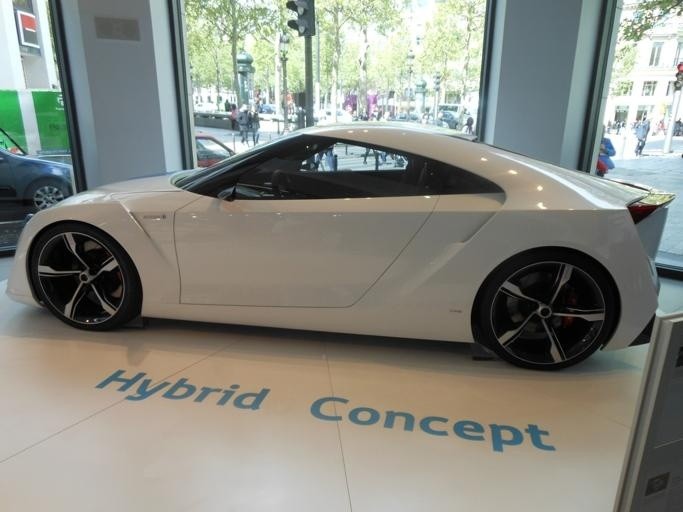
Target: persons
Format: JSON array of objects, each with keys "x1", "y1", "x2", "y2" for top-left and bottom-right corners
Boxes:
[
  {"x1": 596, "y1": 125, "x2": 616, "y2": 176},
  {"x1": 224, "y1": 99, "x2": 263, "y2": 148},
  {"x1": 605, "y1": 113, "x2": 683, "y2": 156},
  {"x1": 294, "y1": 105, "x2": 475, "y2": 172}
]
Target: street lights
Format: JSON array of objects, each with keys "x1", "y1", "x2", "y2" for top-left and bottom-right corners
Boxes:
[
  {"x1": 404, "y1": 50, "x2": 415, "y2": 114},
  {"x1": 280, "y1": 30, "x2": 290, "y2": 129}
]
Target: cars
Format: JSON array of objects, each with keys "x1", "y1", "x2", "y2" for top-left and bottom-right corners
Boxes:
[
  {"x1": 193, "y1": 133, "x2": 235, "y2": 168},
  {"x1": 0, "y1": 148, "x2": 73, "y2": 211},
  {"x1": 440, "y1": 112, "x2": 463, "y2": 130},
  {"x1": 386, "y1": 114, "x2": 421, "y2": 123}
]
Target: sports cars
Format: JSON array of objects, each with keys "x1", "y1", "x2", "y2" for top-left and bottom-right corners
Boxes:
[{"x1": 7, "y1": 121, "x2": 675, "y2": 371}]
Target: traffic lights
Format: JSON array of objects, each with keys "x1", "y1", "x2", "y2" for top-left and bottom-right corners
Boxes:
[
  {"x1": 286, "y1": 0, "x2": 315, "y2": 36},
  {"x1": 673, "y1": 62, "x2": 683, "y2": 90}
]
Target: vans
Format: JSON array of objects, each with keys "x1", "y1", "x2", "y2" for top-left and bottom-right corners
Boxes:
[{"x1": 428, "y1": 103, "x2": 463, "y2": 119}]
